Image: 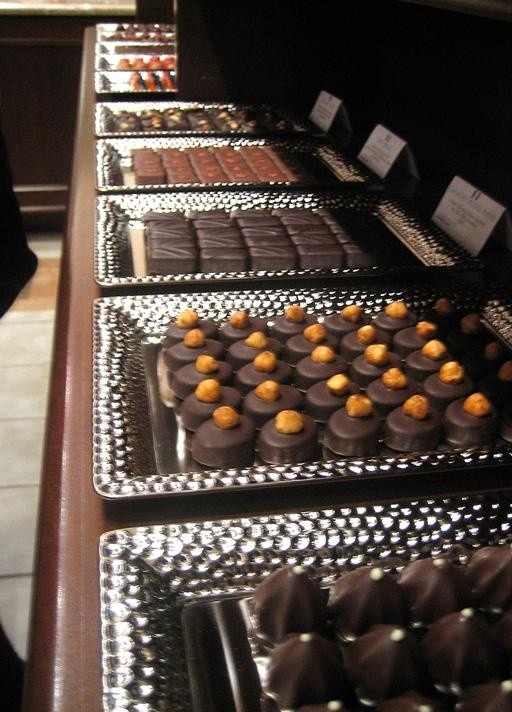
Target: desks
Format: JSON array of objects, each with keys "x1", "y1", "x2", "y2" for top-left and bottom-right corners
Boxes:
[{"x1": 26, "y1": 27, "x2": 512, "y2": 712}]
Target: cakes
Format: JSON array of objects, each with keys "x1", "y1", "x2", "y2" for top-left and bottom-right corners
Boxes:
[
  {"x1": 130, "y1": 145, "x2": 316, "y2": 184},
  {"x1": 144, "y1": 206, "x2": 371, "y2": 275},
  {"x1": 112, "y1": 22, "x2": 177, "y2": 92},
  {"x1": 114, "y1": 106, "x2": 290, "y2": 134}
]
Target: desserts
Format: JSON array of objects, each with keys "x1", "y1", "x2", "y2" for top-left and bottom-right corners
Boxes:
[
  {"x1": 251, "y1": 546, "x2": 512, "y2": 712},
  {"x1": 163, "y1": 297, "x2": 512, "y2": 469}
]
